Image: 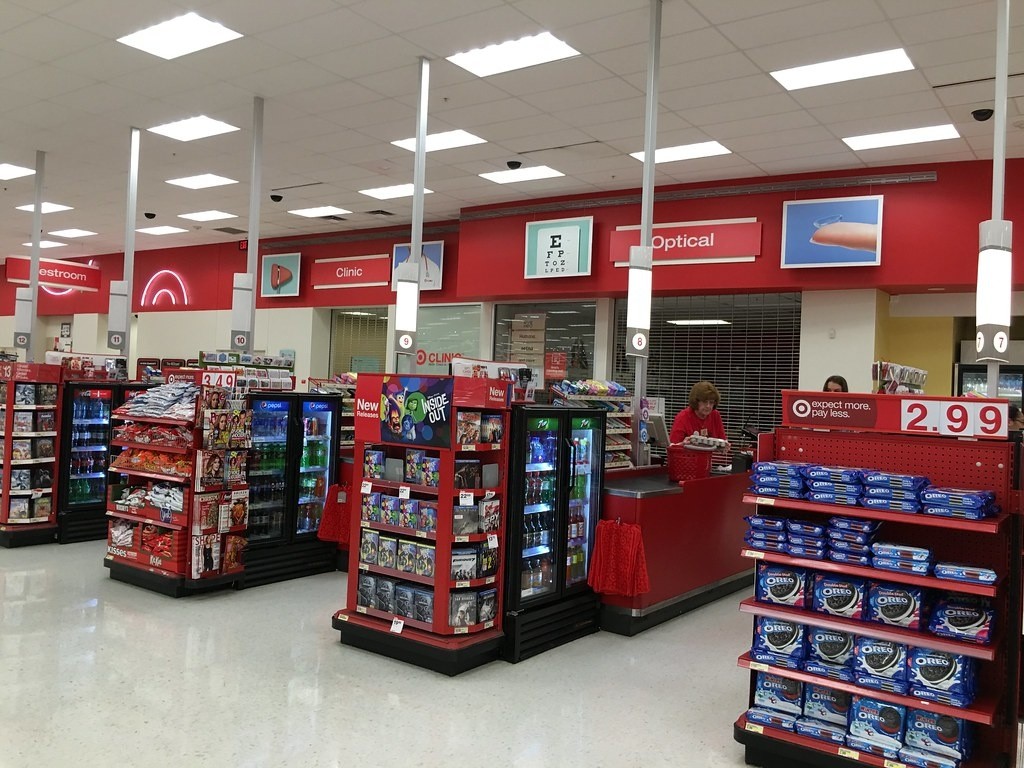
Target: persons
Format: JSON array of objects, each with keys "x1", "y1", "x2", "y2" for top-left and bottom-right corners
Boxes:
[
  {"x1": 230, "y1": 457, "x2": 236, "y2": 467},
  {"x1": 232, "y1": 415, "x2": 239, "y2": 423},
  {"x1": 238, "y1": 410, "x2": 247, "y2": 432},
  {"x1": 209, "y1": 392, "x2": 219, "y2": 409},
  {"x1": 213, "y1": 414, "x2": 227, "y2": 432},
  {"x1": 1007, "y1": 406, "x2": 1024, "y2": 431},
  {"x1": 802, "y1": 375, "x2": 854, "y2": 433},
  {"x1": 205, "y1": 454, "x2": 222, "y2": 476},
  {"x1": 201, "y1": 535, "x2": 214, "y2": 571},
  {"x1": 669, "y1": 381, "x2": 731, "y2": 455},
  {"x1": 236, "y1": 451, "x2": 242, "y2": 474}
]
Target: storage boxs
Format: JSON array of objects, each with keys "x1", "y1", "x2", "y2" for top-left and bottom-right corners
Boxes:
[
  {"x1": 754, "y1": 563, "x2": 997, "y2": 761},
  {"x1": 509, "y1": 313, "x2": 547, "y2": 389}
]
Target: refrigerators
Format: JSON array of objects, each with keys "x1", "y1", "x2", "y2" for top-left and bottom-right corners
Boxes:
[
  {"x1": 241, "y1": 394, "x2": 344, "y2": 588},
  {"x1": 55, "y1": 383, "x2": 161, "y2": 542},
  {"x1": 495, "y1": 403, "x2": 602, "y2": 663}
]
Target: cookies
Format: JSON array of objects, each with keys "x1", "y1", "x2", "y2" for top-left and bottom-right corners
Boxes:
[{"x1": 765, "y1": 571, "x2": 987, "y2": 684}]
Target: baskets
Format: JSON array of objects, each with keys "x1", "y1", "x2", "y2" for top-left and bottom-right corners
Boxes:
[{"x1": 666, "y1": 443, "x2": 717, "y2": 481}]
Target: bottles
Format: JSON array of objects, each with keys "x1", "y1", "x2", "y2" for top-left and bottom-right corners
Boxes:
[
  {"x1": 251, "y1": 416, "x2": 325, "y2": 536},
  {"x1": 68, "y1": 399, "x2": 127, "y2": 506},
  {"x1": 520, "y1": 429, "x2": 591, "y2": 593}
]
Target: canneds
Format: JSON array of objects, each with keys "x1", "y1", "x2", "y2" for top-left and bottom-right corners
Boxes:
[{"x1": 303, "y1": 416, "x2": 320, "y2": 437}]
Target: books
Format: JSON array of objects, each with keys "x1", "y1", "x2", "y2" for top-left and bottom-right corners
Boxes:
[
  {"x1": 0, "y1": 383, "x2": 57, "y2": 520},
  {"x1": 191, "y1": 390, "x2": 251, "y2": 579},
  {"x1": 449, "y1": 411, "x2": 504, "y2": 626}
]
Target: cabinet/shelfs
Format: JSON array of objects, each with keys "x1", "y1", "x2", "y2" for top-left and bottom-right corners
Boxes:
[
  {"x1": 550, "y1": 384, "x2": 636, "y2": 468},
  {"x1": 0, "y1": 360, "x2": 64, "y2": 550},
  {"x1": 328, "y1": 374, "x2": 512, "y2": 678},
  {"x1": 102, "y1": 386, "x2": 252, "y2": 597},
  {"x1": 744, "y1": 389, "x2": 1024, "y2": 768}
]
